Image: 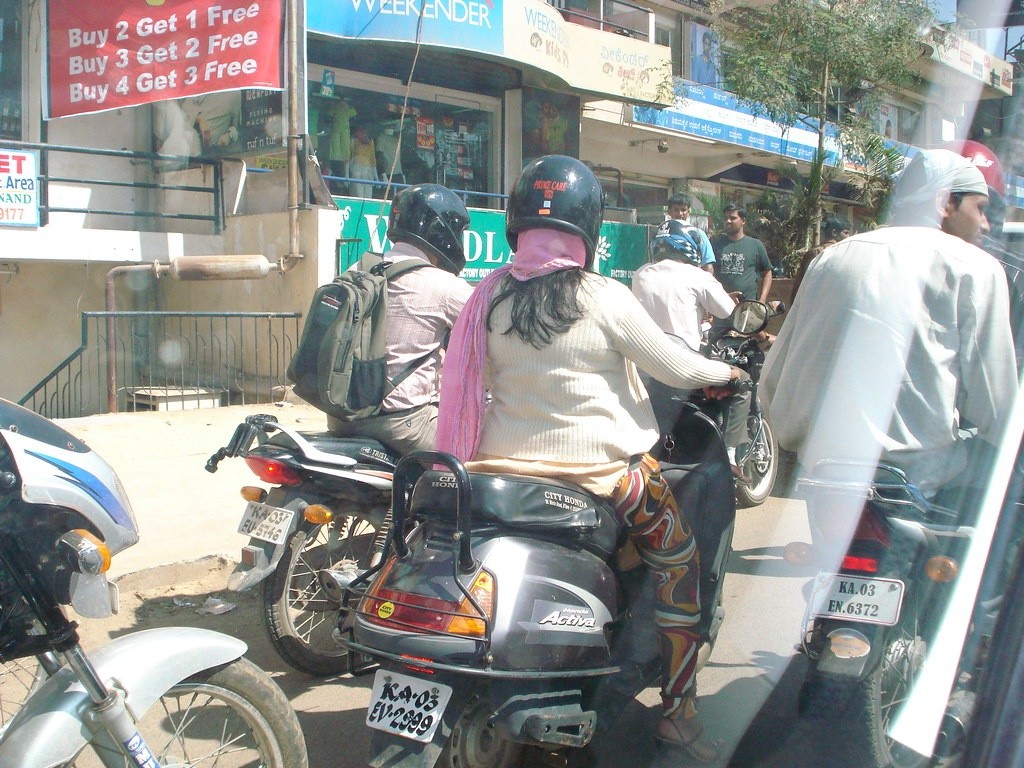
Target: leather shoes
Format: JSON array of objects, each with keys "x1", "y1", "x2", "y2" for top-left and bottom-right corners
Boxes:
[{"x1": 730, "y1": 463, "x2": 753, "y2": 486}]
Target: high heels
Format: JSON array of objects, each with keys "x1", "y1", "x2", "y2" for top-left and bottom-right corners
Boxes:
[{"x1": 653, "y1": 713, "x2": 731, "y2": 764}]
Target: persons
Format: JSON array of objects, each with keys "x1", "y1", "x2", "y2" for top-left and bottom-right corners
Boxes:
[
  {"x1": 631, "y1": 140, "x2": 1023, "y2": 497},
  {"x1": 434, "y1": 152, "x2": 751, "y2": 765},
  {"x1": 327, "y1": 184, "x2": 476, "y2": 472},
  {"x1": 309, "y1": 100, "x2": 405, "y2": 199},
  {"x1": 691, "y1": 32, "x2": 719, "y2": 87}
]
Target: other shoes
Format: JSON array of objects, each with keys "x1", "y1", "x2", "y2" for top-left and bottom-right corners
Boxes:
[{"x1": 798, "y1": 681, "x2": 847, "y2": 722}]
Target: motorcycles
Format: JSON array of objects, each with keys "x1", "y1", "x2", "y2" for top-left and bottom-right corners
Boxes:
[
  {"x1": 354, "y1": 299, "x2": 771, "y2": 763},
  {"x1": 793, "y1": 454, "x2": 1023, "y2": 768},
  {"x1": 212, "y1": 411, "x2": 409, "y2": 679},
  {"x1": 683, "y1": 299, "x2": 785, "y2": 506},
  {"x1": 0, "y1": 399, "x2": 309, "y2": 766}
]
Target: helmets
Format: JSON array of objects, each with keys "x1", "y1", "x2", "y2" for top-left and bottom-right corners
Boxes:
[
  {"x1": 648, "y1": 219, "x2": 704, "y2": 266},
  {"x1": 505, "y1": 154, "x2": 606, "y2": 270},
  {"x1": 386, "y1": 182, "x2": 472, "y2": 277},
  {"x1": 933, "y1": 139, "x2": 1005, "y2": 204}
]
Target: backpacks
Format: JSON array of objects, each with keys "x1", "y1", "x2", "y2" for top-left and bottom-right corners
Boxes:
[{"x1": 287, "y1": 249, "x2": 438, "y2": 422}]
